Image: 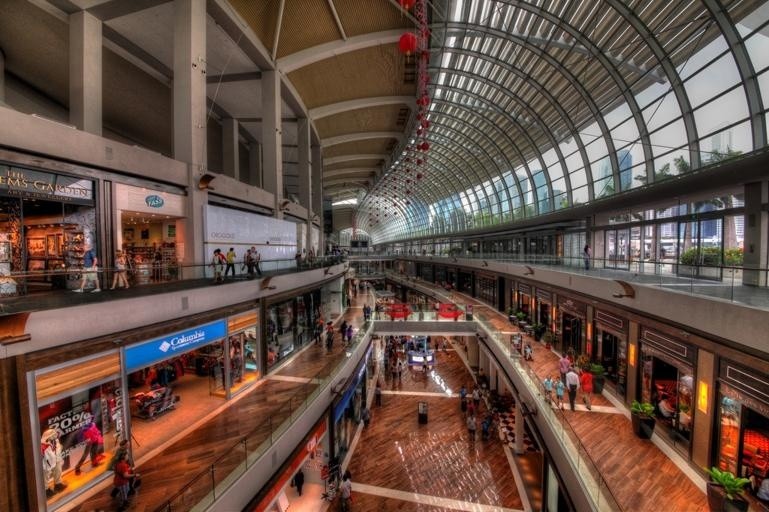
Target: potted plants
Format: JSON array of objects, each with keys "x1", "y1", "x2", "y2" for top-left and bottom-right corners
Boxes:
[
  {"x1": 588, "y1": 357, "x2": 607, "y2": 393},
  {"x1": 696, "y1": 457, "x2": 752, "y2": 512},
  {"x1": 675, "y1": 245, "x2": 730, "y2": 282},
  {"x1": 629, "y1": 394, "x2": 662, "y2": 441}
]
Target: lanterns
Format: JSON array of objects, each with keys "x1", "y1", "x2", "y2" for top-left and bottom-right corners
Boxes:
[{"x1": 368, "y1": 0, "x2": 430, "y2": 228}]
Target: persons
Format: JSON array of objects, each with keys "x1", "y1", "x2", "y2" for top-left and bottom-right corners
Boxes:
[
  {"x1": 432, "y1": 279, "x2": 457, "y2": 294},
  {"x1": 658, "y1": 394, "x2": 675, "y2": 419},
  {"x1": 218, "y1": 334, "x2": 256, "y2": 388},
  {"x1": 327, "y1": 463, "x2": 354, "y2": 512},
  {"x1": 295, "y1": 318, "x2": 353, "y2": 353},
  {"x1": 294, "y1": 245, "x2": 346, "y2": 272},
  {"x1": 407, "y1": 274, "x2": 424, "y2": 284},
  {"x1": 510, "y1": 332, "x2": 533, "y2": 361},
  {"x1": 267, "y1": 345, "x2": 284, "y2": 368},
  {"x1": 543, "y1": 347, "x2": 593, "y2": 412},
  {"x1": 375, "y1": 383, "x2": 382, "y2": 407},
  {"x1": 680, "y1": 371, "x2": 693, "y2": 390},
  {"x1": 582, "y1": 244, "x2": 592, "y2": 275},
  {"x1": 756, "y1": 470, "x2": 769, "y2": 507},
  {"x1": 74, "y1": 413, "x2": 103, "y2": 476},
  {"x1": 294, "y1": 469, "x2": 304, "y2": 496},
  {"x1": 51, "y1": 244, "x2": 162, "y2": 293},
  {"x1": 346, "y1": 287, "x2": 352, "y2": 307},
  {"x1": 38, "y1": 428, "x2": 67, "y2": 498},
  {"x1": 363, "y1": 295, "x2": 411, "y2": 323},
  {"x1": 459, "y1": 368, "x2": 515, "y2": 442},
  {"x1": 382, "y1": 334, "x2": 447, "y2": 380},
  {"x1": 644, "y1": 354, "x2": 652, "y2": 392},
  {"x1": 210, "y1": 246, "x2": 263, "y2": 282},
  {"x1": 107, "y1": 439, "x2": 140, "y2": 512},
  {"x1": 359, "y1": 400, "x2": 369, "y2": 430}
]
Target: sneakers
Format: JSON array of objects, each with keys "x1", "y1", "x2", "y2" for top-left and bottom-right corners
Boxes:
[{"x1": 71, "y1": 288, "x2": 101, "y2": 293}]
